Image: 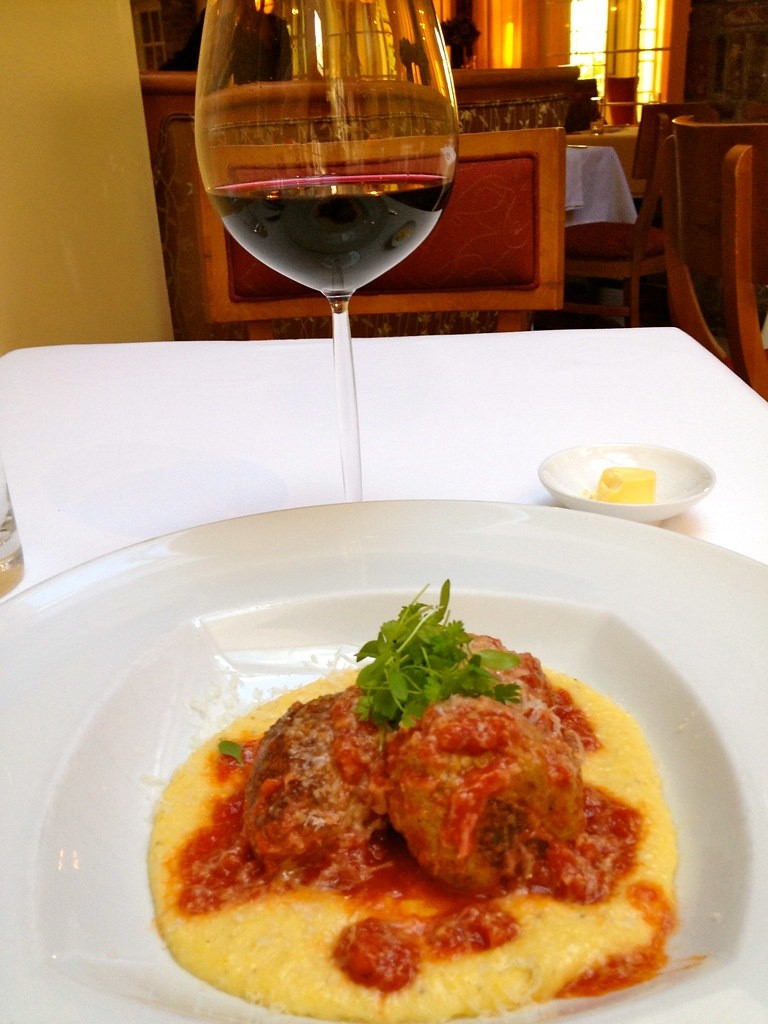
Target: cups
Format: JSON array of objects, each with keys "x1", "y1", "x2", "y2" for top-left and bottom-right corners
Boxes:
[
  {"x1": 587, "y1": 97, "x2": 605, "y2": 137},
  {"x1": 1, "y1": 455, "x2": 24, "y2": 599}
]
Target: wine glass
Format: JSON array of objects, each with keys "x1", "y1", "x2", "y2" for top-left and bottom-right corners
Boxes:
[{"x1": 193, "y1": 0, "x2": 458, "y2": 500}]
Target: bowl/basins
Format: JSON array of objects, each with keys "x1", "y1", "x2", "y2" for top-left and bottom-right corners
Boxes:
[
  {"x1": 1, "y1": 497, "x2": 767, "y2": 1024},
  {"x1": 538, "y1": 444, "x2": 720, "y2": 523}
]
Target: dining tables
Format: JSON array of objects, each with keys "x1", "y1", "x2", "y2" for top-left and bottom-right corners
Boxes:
[{"x1": 0, "y1": 326, "x2": 768, "y2": 1024}]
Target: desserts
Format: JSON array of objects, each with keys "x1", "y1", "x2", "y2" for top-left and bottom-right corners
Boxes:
[{"x1": 595, "y1": 466, "x2": 658, "y2": 504}]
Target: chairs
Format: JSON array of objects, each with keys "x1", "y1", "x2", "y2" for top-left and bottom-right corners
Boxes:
[{"x1": 136, "y1": 67, "x2": 768, "y2": 392}]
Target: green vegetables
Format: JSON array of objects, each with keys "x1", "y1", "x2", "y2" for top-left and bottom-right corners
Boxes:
[
  {"x1": 353, "y1": 579, "x2": 522, "y2": 729},
  {"x1": 218, "y1": 741, "x2": 243, "y2": 764}
]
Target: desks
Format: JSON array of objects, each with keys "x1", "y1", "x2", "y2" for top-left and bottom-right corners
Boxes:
[{"x1": 567, "y1": 122, "x2": 649, "y2": 196}]
